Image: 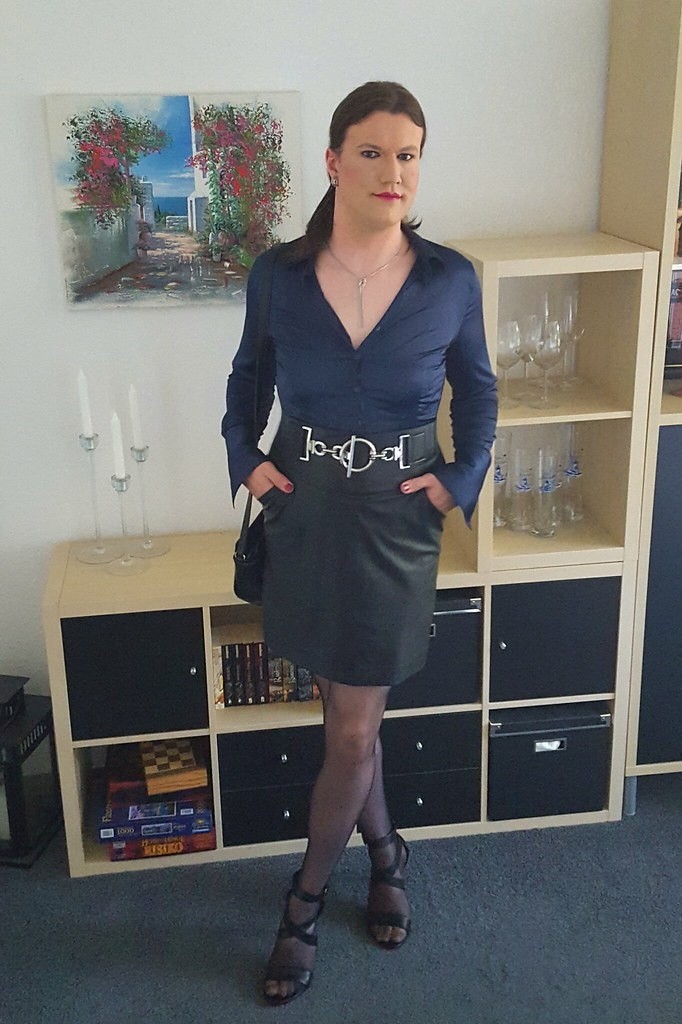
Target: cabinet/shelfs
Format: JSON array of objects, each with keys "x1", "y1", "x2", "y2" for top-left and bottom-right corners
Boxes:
[
  {"x1": 601, "y1": 0, "x2": 682, "y2": 811},
  {"x1": 42, "y1": 231, "x2": 659, "y2": 882}
]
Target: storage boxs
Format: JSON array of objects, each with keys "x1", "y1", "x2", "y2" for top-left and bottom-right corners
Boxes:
[
  {"x1": 384, "y1": 589, "x2": 483, "y2": 712},
  {"x1": 488, "y1": 702, "x2": 610, "y2": 823},
  {"x1": 386, "y1": 592, "x2": 480, "y2": 710},
  {"x1": 96, "y1": 738, "x2": 216, "y2": 863}
]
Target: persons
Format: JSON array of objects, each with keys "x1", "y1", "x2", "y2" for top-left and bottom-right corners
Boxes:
[{"x1": 221, "y1": 82, "x2": 498, "y2": 1007}]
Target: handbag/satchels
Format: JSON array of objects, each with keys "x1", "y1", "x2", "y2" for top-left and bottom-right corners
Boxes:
[{"x1": 232, "y1": 509, "x2": 265, "y2": 605}]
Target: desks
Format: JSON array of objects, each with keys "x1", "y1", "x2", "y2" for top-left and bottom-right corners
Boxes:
[{"x1": 0, "y1": 695, "x2": 62, "y2": 870}]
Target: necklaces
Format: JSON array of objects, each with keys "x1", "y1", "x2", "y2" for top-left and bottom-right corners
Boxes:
[{"x1": 327, "y1": 236, "x2": 406, "y2": 328}]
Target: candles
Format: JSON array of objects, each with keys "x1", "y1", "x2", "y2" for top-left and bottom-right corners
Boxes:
[
  {"x1": 109, "y1": 413, "x2": 125, "y2": 479},
  {"x1": 127, "y1": 383, "x2": 144, "y2": 449},
  {"x1": 77, "y1": 368, "x2": 93, "y2": 437}
]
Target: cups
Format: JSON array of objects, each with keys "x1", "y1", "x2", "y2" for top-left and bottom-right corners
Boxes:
[{"x1": 495, "y1": 423, "x2": 585, "y2": 538}]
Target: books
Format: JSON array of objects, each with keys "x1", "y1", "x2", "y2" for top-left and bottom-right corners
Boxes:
[
  {"x1": 211, "y1": 622, "x2": 321, "y2": 709},
  {"x1": 86, "y1": 738, "x2": 218, "y2": 861}
]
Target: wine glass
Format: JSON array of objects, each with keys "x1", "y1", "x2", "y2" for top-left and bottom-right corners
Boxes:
[{"x1": 497, "y1": 286, "x2": 584, "y2": 411}]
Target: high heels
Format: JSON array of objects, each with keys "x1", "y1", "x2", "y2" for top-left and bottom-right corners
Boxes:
[
  {"x1": 263, "y1": 871, "x2": 329, "y2": 1006},
  {"x1": 364, "y1": 816, "x2": 412, "y2": 949}
]
